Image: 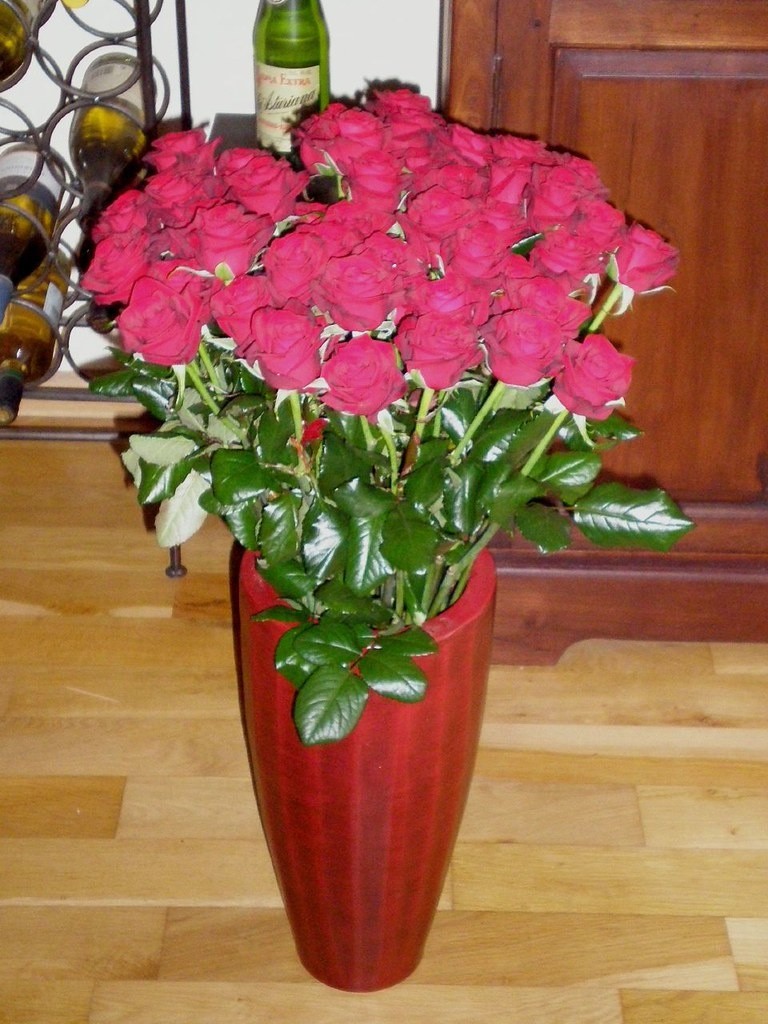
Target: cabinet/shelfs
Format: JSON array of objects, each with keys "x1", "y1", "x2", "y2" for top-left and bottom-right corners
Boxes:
[{"x1": 435, "y1": 0, "x2": 768, "y2": 665}]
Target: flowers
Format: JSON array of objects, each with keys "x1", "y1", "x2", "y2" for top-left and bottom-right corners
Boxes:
[{"x1": 80, "y1": 90, "x2": 697, "y2": 747}]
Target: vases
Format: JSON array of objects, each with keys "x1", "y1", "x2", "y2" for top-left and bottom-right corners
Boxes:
[{"x1": 236, "y1": 546, "x2": 496, "y2": 993}]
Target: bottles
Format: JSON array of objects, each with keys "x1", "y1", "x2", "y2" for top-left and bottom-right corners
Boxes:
[
  {"x1": 0, "y1": 0, "x2": 53, "y2": 84},
  {"x1": 0, "y1": 141, "x2": 64, "y2": 329},
  {"x1": 66, "y1": 50, "x2": 143, "y2": 237},
  {"x1": 252, "y1": 0, "x2": 332, "y2": 172},
  {"x1": 0, "y1": 249, "x2": 73, "y2": 428}
]
